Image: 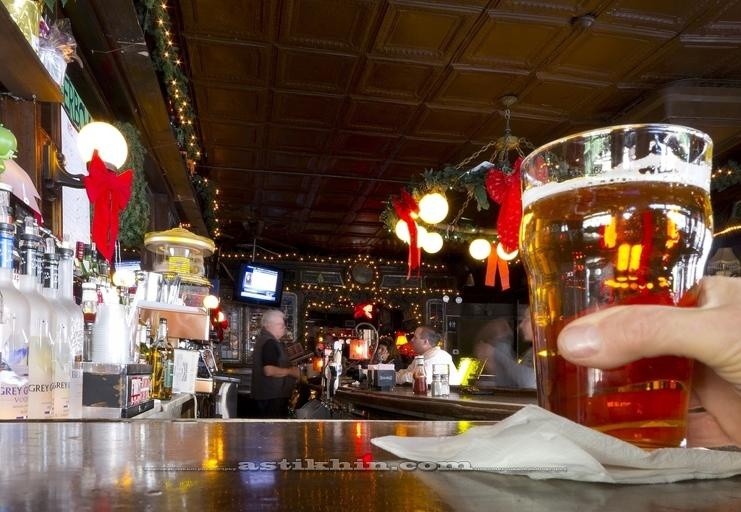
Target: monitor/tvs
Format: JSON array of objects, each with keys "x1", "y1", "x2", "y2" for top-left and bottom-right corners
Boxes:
[{"x1": 232, "y1": 260, "x2": 286, "y2": 308}]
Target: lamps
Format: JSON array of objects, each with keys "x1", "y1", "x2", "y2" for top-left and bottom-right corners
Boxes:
[
  {"x1": 348, "y1": 321, "x2": 381, "y2": 366},
  {"x1": 379, "y1": 95, "x2": 592, "y2": 264},
  {"x1": 42, "y1": 120, "x2": 131, "y2": 203}
]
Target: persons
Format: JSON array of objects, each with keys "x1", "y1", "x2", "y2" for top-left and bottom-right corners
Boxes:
[
  {"x1": 361, "y1": 325, "x2": 461, "y2": 389},
  {"x1": 247, "y1": 309, "x2": 303, "y2": 418},
  {"x1": 555, "y1": 266, "x2": 741, "y2": 456},
  {"x1": 373, "y1": 336, "x2": 408, "y2": 387},
  {"x1": 473, "y1": 302, "x2": 537, "y2": 393}
]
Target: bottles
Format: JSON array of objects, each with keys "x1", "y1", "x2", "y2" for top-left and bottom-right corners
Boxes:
[
  {"x1": 1, "y1": 201, "x2": 131, "y2": 422},
  {"x1": 136, "y1": 317, "x2": 174, "y2": 400},
  {"x1": 413, "y1": 355, "x2": 428, "y2": 395}
]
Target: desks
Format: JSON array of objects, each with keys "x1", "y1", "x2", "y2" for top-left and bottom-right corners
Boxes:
[{"x1": 0, "y1": 417, "x2": 741, "y2": 511}]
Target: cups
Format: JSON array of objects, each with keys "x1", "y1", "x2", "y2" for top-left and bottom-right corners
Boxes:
[
  {"x1": 517, "y1": 121, "x2": 716, "y2": 450},
  {"x1": 432, "y1": 362, "x2": 451, "y2": 397}
]
